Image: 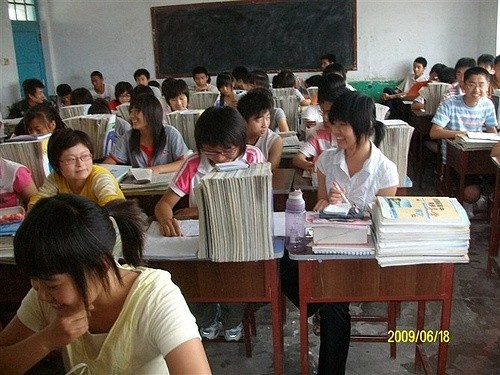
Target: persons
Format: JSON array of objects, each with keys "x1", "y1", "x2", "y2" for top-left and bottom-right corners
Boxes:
[
  {"x1": 0, "y1": 193, "x2": 211, "y2": 375},
  {"x1": 0, "y1": 157, "x2": 39, "y2": 209},
  {"x1": 0, "y1": 53, "x2": 500, "y2": 172},
  {"x1": 277, "y1": 91, "x2": 400, "y2": 375},
  {"x1": 155, "y1": 106, "x2": 266, "y2": 342},
  {"x1": 27, "y1": 128, "x2": 127, "y2": 206}
]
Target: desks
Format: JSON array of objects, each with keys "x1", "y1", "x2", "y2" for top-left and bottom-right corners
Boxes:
[{"x1": 0, "y1": 96, "x2": 500, "y2": 375}]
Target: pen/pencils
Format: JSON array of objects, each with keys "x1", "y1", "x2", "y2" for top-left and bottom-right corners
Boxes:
[{"x1": 333, "y1": 182, "x2": 349, "y2": 203}]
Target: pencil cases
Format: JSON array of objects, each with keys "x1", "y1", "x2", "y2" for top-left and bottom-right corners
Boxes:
[{"x1": 319, "y1": 204, "x2": 363, "y2": 219}]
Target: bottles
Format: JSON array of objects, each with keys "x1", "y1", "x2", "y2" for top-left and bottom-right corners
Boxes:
[
  {"x1": 284, "y1": 185, "x2": 306, "y2": 254},
  {"x1": 306, "y1": 120, "x2": 316, "y2": 140}
]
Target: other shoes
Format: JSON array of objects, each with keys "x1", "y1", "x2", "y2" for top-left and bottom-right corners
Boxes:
[
  {"x1": 313, "y1": 308, "x2": 321, "y2": 335},
  {"x1": 463, "y1": 202, "x2": 474, "y2": 220}
]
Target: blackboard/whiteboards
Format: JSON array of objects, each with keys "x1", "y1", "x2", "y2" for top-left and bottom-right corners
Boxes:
[{"x1": 151, "y1": 0, "x2": 357, "y2": 79}]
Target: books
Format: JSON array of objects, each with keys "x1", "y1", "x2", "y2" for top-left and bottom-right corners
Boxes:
[{"x1": 0, "y1": 82, "x2": 500, "y2": 269}]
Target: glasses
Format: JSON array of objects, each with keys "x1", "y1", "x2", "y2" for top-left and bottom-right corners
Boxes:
[
  {"x1": 203, "y1": 146, "x2": 237, "y2": 156},
  {"x1": 59, "y1": 154, "x2": 93, "y2": 165}
]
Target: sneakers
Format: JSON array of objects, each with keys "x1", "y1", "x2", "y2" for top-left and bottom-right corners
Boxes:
[
  {"x1": 223, "y1": 322, "x2": 243, "y2": 342},
  {"x1": 199, "y1": 321, "x2": 220, "y2": 340}
]
